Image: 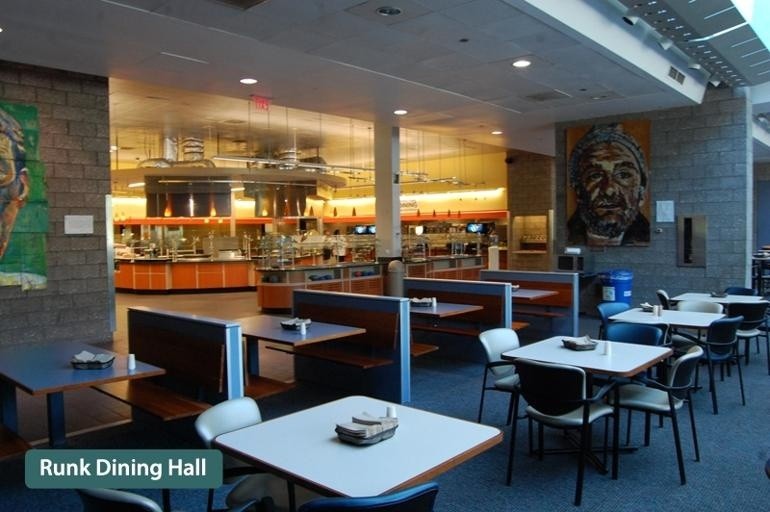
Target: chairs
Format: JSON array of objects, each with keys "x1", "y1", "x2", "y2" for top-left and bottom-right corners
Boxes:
[{"x1": 0, "y1": 285, "x2": 770, "y2": 512}]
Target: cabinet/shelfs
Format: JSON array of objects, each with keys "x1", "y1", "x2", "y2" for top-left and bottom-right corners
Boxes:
[{"x1": 254, "y1": 255, "x2": 485, "y2": 313}]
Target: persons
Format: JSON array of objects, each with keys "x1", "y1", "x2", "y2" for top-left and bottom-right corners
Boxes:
[
  {"x1": 323, "y1": 229, "x2": 330, "y2": 260},
  {"x1": 0, "y1": 107, "x2": 30, "y2": 259},
  {"x1": 565, "y1": 124, "x2": 650, "y2": 247},
  {"x1": 332, "y1": 229, "x2": 347, "y2": 262}
]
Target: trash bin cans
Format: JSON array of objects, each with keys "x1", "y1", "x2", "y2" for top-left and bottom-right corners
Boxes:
[{"x1": 598, "y1": 269, "x2": 634, "y2": 309}]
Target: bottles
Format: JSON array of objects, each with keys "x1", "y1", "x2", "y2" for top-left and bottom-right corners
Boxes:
[
  {"x1": 432, "y1": 297, "x2": 437, "y2": 310},
  {"x1": 387, "y1": 406, "x2": 397, "y2": 418},
  {"x1": 605, "y1": 341, "x2": 611, "y2": 355},
  {"x1": 300, "y1": 322, "x2": 307, "y2": 336},
  {"x1": 653, "y1": 304, "x2": 664, "y2": 318},
  {"x1": 127, "y1": 353, "x2": 137, "y2": 371}
]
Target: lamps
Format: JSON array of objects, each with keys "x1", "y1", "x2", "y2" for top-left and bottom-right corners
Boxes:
[
  {"x1": 213, "y1": 107, "x2": 461, "y2": 219},
  {"x1": 708, "y1": 73, "x2": 722, "y2": 86},
  {"x1": 622, "y1": 5, "x2": 641, "y2": 25},
  {"x1": 687, "y1": 56, "x2": 703, "y2": 71},
  {"x1": 657, "y1": 34, "x2": 674, "y2": 51}
]
